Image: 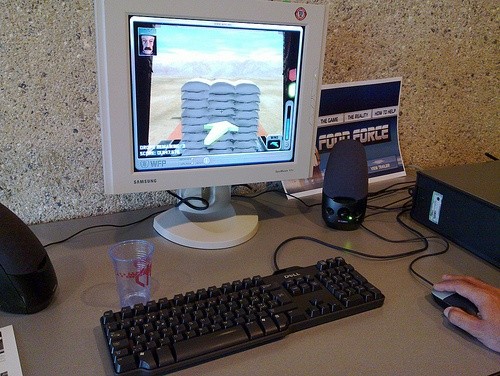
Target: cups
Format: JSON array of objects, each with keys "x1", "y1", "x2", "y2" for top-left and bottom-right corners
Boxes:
[{"x1": 109, "y1": 240, "x2": 154, "y2": 308}]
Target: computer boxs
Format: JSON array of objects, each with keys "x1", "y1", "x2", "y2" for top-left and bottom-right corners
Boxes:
[{"x1": 413, "y1": 159, "x2": 500, "y2": 267}]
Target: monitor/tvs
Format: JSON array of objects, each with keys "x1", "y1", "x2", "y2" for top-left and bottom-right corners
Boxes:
[{"x1": 91, "y1": 1, "x2": 327, "y2": 249}]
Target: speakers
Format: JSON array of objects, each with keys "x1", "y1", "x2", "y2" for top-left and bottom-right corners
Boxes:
[
  {"x1": 321, "y1": 140, "x2": 367, "y2": 230},
  {"x1": 0, "y1": 203, "x2": 56, "y2": 313}
]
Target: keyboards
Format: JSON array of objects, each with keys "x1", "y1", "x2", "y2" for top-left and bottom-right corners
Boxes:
[{"x1": 99, "y1": 256, "x2": 386, "y2": 375}]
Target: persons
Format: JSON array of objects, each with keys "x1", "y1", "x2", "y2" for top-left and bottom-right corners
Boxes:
[{"x1": 433, "y1": 273, "x2": 500, "y2": 354}]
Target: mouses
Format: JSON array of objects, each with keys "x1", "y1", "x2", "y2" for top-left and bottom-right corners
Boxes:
[{"x1": 431, "y1": 289, "x2": 481, "y2": 317}]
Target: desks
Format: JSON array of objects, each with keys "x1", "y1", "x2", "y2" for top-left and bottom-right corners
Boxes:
[{"x1": 0, "y1": 169, "x2": 500, "y2": 376}]
